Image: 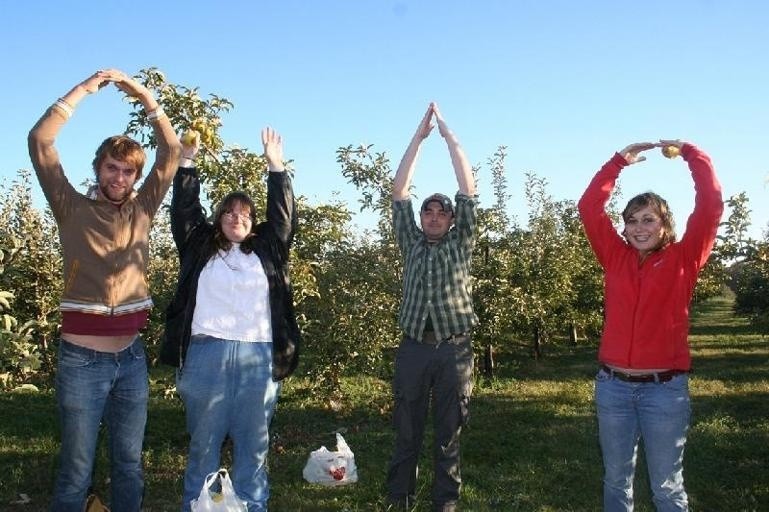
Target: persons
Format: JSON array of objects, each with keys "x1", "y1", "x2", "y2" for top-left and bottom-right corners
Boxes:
[
  {"x1": 26, "y1": 69, "x2": 184, "y2": 511},
  {"x1": 159, "y1": 125, "x2": 301, "y2": 511},
  {"x1": 384, "y1": 101, "x2": 481, "y2": 511},
  {"x1": 578, "y1": 138, "x2": 724, "y2": 512}
]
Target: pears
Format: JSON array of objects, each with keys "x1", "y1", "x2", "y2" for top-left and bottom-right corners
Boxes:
[
  {"x1": 180, "y1": 120, "x2": 213, "y2": 146},
  {"x1": 662, "y1": 146, "x2": 680, "y2": 158}
]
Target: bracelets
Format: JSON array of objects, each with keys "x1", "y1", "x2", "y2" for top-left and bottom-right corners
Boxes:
[{"x1": 180, "y1": 155, "x2": 195, "y2": 162}]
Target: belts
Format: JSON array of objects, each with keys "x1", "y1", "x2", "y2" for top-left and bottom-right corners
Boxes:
[
  {"x1": 401, "y1": 331, "x2": 467, "y2": 345},
  {"x1": 601, "y1": 364, "x2": 690, "y2": 384}
]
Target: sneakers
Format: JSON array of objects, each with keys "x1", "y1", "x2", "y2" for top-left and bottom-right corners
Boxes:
[{"x1": 384, "y1": 498, "x2": 457, "y2": 512}]
